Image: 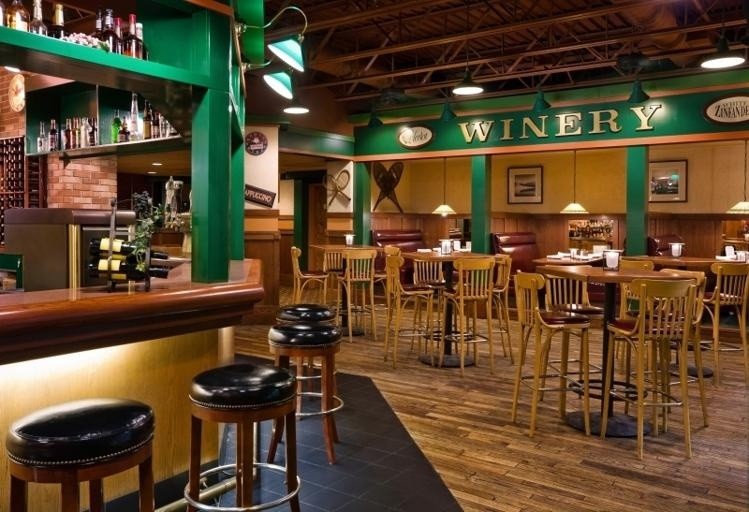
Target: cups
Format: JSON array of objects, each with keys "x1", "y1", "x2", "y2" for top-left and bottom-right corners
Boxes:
[
  {"x1": 453, "y1": 241, "x2": 461, "y2": 251},
  {"x1": 580, "y1": 250, "x2": 588, "y2": 259},
  {"x1": 466, "y1": 242, "x2": 472, "y2": 251}
]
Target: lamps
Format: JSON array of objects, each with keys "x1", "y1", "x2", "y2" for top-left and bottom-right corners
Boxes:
[
  {"x1": 238, "y1": 3, "x2": 314, "y2": 116},
  {"x1": 701, "y1": 27, "x2": 746, "y2": 73},
  {"x1": 726, "y1": 137, "x2": 749, "y2": 217},
  {"x1": 365, "y1": 74, "x2": 650, "y2": 128},
  {"x1": 432, "y1": 157, "x2": 456, "y2": 218},
  {"x1": 559, "y1": 150, "x2": 592, "y2": 216},
  {"x1": 452, "y1": 1, "x2": 486, "y2": 97}
]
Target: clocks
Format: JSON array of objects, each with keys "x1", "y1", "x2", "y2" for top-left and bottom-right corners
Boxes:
[{"x1": 7, "y1": 76, "x2": 28, "y2": 114}]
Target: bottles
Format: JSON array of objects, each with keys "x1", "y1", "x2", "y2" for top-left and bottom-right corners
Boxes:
[
  {"x1": 568, "y1": 219, "x2": 613, "y2": 240},
  {"x1": 36, "y1": 90, "x2": 180, "y2": 152},
  {"x1": 85, "y1": 238, "x2": 170, "y2": 281},
  {"x1": 0, "y1": 0, "x2": 149, "y2": 62}
]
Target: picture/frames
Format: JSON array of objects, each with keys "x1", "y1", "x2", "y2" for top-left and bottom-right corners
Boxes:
[
  {"x1": 506, "y1": 165, "x2": 543, "y2": 205},
  {"x1": 647, "y1": 159, "x2": 689, "y2": 204}
]
